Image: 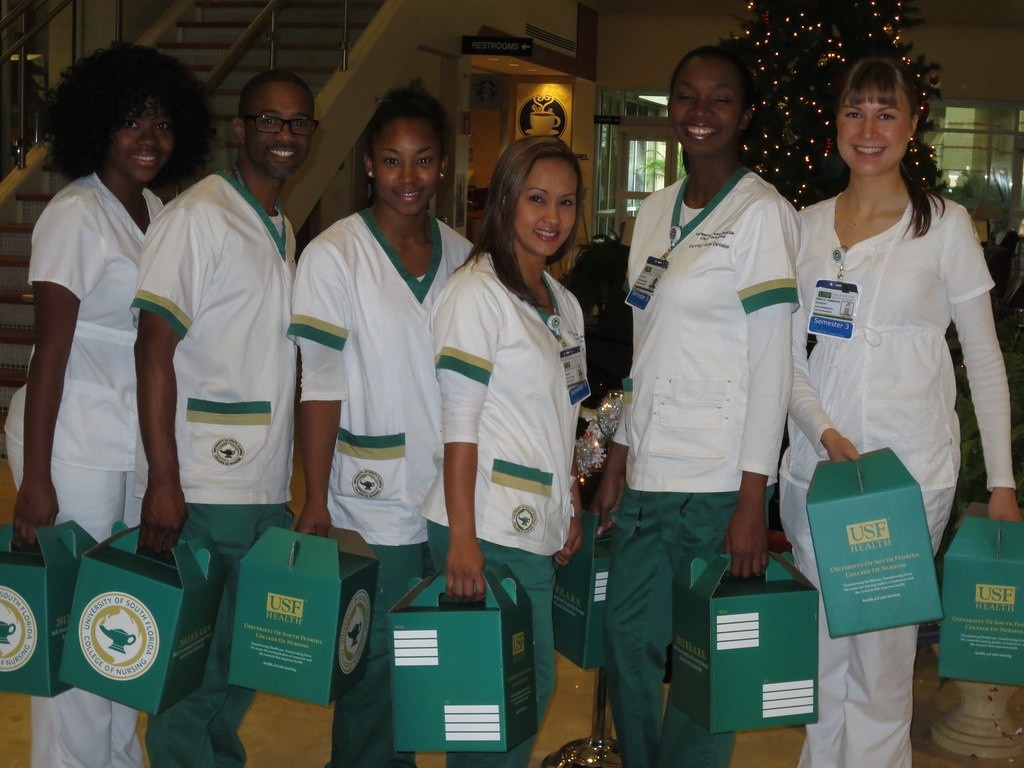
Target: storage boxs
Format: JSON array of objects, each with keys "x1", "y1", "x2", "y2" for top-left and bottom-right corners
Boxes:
[
  {"x1": 387, "y1": 565, "x2": 539, "y2": 753},
  {"x1": 0, "y1": 521, "x2": 99, "y2": 698},
  {"x1": 805, "y1": 446, "x2": 944, "y2": 637},
  {"x1": 554, "y1": 508, "x2": 616, "y2": 672},
  {"x1": 58, "y1": 526, "x2": 227, "y2": 716},
  {"x1": 228, "y1": 528, "x2": 378, "y2": 707},
  {"x1": 938, "y1": 516, "x2": 1024, "y2": 688},
  {"x1": 672, "y1": 549, "x2": 819, "y2": 733}
]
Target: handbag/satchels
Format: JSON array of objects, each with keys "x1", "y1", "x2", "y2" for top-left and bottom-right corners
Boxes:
[
  {"x1": 806, "y1": 448, "x2": 944, "y2": 639},
  {"x1": 671, "y1": 553, "x2": 819, "y2": 734},
  {"x1": 387, "y1": 563, "x2": 539, "y2": 751},
  {"x1": 0, "y1": 520, "x2": 228, "y2": 716},
  {"x1": 937, "y1": 502, "x2": 1024, "y2": 688},
  {"x1": 552, "y1": 508, "x2": 618, "y2": 670},
  {"x1": 228, "y1": 525, "x2": 380, "y2": 709}
]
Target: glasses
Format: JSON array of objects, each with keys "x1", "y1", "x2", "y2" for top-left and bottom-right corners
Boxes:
[{"x1": 242, "y1": 115, "x2": 319, "y2": 136}]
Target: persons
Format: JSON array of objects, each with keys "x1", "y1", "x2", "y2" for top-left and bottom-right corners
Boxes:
[
  {"x1": 133, "y1": 71, "x2": 323, "y2": 758},
  {"x1": 590, "y1": 73, "x2": 801, "y2": 764},
  {"x1": 5, "y1": 43, "x2": 207, "y2": 767},
  {"x1": 786, "y1": 50, "x2": 1016, "y2": 767},
  {"x1": 284, "y1": 89, "x2": 474, "y2": 761},
  {"x1": 430, "y1": 133, "x2": 590, "y2": 758}
]
